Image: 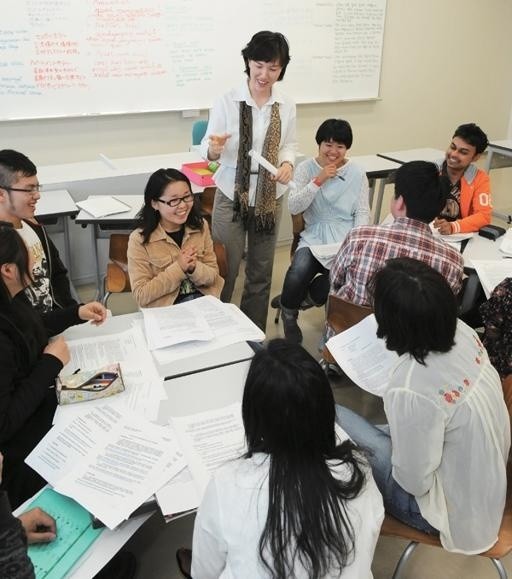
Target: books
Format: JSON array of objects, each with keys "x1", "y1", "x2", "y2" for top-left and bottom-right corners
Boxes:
[
  {"x1": 75, "y1": 196, "x2": 132, "y2": 219},
  {"x1": 152, "y1": 467, "x2": 199, "y2": 523}
]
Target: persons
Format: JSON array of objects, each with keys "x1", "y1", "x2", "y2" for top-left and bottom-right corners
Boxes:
[
  {"x1": 334, "y1": 256, "x2": 512, "y2": 555},
  {"x1": 432, "y1": 121, "x2": 492, "y2": 235},
  {"x1": 0, "y1": 222, "x2": 107, "y2": 490},
  {"x1": 0, "y1": 149, "x2": 77, "y2": 323},
  {"x1": 271, "y1": 119, "x2": 371, "y2": 345},
  {"x1": 0, "y1": 450, "x2": 134, "y2": 578},
  {"x1": 176, "y1": 341, "x2": 385, "y2": 579},
  {"x1": 127, "y1": 169, "x2": 224, "y2": 308},
  {"x1": 200, "y1": 31, "x2": 298, "y2": 332},
  {"x1": 319, "y1": 161, "x2": 465, "y2": 376}
]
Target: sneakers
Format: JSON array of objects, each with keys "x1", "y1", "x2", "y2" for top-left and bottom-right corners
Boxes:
[{"x1": 98, "y1": 551, "x2": 137, "y2": 579}]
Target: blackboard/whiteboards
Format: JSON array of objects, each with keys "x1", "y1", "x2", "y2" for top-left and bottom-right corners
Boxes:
[{"x1": 2, "y1": 2, "x2": 387, "y2": 124}]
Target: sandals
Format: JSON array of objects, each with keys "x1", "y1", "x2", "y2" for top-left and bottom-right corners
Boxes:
[{"x1": 175, "y1": 547, "x2": 192, "y2": 579}]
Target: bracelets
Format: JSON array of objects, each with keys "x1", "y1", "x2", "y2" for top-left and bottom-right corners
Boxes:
[{"x1": 311, "y1": 175, "x2": 321, "y2": 186}]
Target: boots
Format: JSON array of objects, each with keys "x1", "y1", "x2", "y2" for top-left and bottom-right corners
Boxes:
[
  {"x1": 271, "y1": 290, "x2": 324, "y2": 311},
  {"x1": 278, "y1": 299, "x2": 303, "y2": 344}
]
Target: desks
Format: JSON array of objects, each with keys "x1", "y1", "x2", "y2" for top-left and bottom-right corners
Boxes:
[
  {"x1": 48, "y1": 294, "x2": 258, "y2": 402},
  {"x1": 75, "y1": 194, "x2": 146, "y2": 301},
  {"x1": 486, "y1": 140, "x2": 512, "y2": 224},
  {"x1": 345, "y1": 155, "x2": 403, "y2": 225},
  {"x1": 377, "y1": 147, "x2": 446, "y2": 171},
  {"x1": 47, "y1": 357, "x2": 357, "y2": 529},
  {"x1": 33, "y1": 189, "x2": 81, "y2": 303}
]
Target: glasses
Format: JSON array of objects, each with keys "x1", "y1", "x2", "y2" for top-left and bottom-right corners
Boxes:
[
  {"x1": 153, "y1": 194, "x2": 194, "y2": 208},
  {"x1": 0, "y1": 184, "x2": 42, "y2": 192}
]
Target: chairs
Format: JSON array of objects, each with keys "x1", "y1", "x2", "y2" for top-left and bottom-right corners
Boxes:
[
  {"x1": 319, "y1": 294, "x2": 374, "y2": 376},
  {"x1": 274, "y1": 213, "x2": 304, "y2": 325},
  {"x1": 101, "y1": 234, "x2": 131, "y2": 308},
  {"x1": 380, "y1": 375, "x2": 511, "y2": 579}
]
